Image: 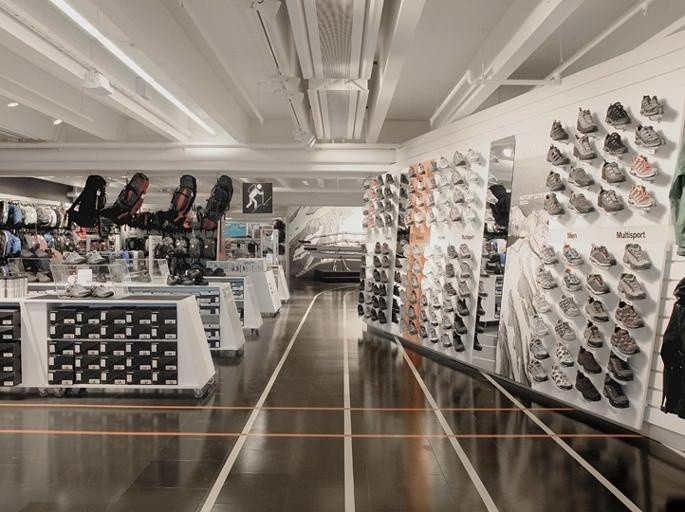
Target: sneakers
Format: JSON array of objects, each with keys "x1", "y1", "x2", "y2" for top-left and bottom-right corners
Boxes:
[
  {"x1": 617, "y1": 272, "x2": 646, "y2": 301},
  {"x1": 578, "y1": 347, "x2": 601, "y2": 373},
  {"x1": 64, "y1": 283, "x2": 91, "y2": 297},
  {"x1": 585, "y1": 273, "x2": 609, "y2": 296},
  {"x1": 165, "y1": 268, "x2": 209, "y2": 285},
  {"x1": 603, "y1": 132, "x2": 628, "y2": 153},
  {"x1": 640, "y1": 94, "x2": 666, "y2": 117},
  {"x1": 606, "y1": 351, "x2": 634, "y2": 381},
  {"x1": 95, "y1": 271, "x2": 122, "y2": 283},
  {"x1": 596, "y1": 188, "x2": 624, "y2": 214},
  {"x1": 585, "y1": 297, "x2": 608, "y2": 323},
  {"x1": 628, "y1": 153, "x2": 658, "y2": 181},
  {"x1": 602, "y1": 161, "x2": 626, "y2": 183},
  {"x1": 635, "y1": 124, "x2": 664, "y2": 146},
  {"x1": 61, "y1": 249, "x2": 106, "y2": 265},
  {"x1": 22, "y1": 271, "x2": 52, "y2": 282},
  {"x1": 622, "y1": 242, "x2": 652, "y2": 271},
  {"x1": 590, "y1": 244, "x2": 617, "y2": 268},
  {"x1": 134, "y1": 270, "x2": 152, "y2": 283},
  {"x1": 357, "y1": 241, "x2": 473, "y2": 353},
  {"x1": 610, "y1": 326, "x2": 638, "y2": 354},
  {"x1": 526, "y1": 244, "x2": 585, "y2": 391},
  {"x1": 582, "y1": 322, "x2": 603, "y2": 348},
  {"x1": 575, "y1": 370, "x2": 601, "y2": 402},
  {"x1": 541, "y1": 105, "x2": 599, "y2": 215},
  {"x1": 604, "y1": 101, "x2": 631, "y2": 127},
  {"x1": 602, "y1": 377, "x2": 629, "y2": 409},
  {"x1": 473, "y1": 150, "x2": 500, "y2": 354},
  {"x1": 628, "y1": 185, "x2": 659, "y2": 210},
  {"x1": 91, "y1": 283, "x2": 115, "y2": 298},
  {"x1": 615, "y1": 301, "x2": 644, "y2": 328}
]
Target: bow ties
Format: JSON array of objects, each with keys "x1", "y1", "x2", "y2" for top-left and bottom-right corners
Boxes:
[{"x1": 360, "y1": 147, "x2": 481, "y2": 230}]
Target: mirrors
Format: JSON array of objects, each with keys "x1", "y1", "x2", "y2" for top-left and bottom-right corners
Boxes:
[{"x1": 467, "y1": 135, "x2": 519, "y2": 372}]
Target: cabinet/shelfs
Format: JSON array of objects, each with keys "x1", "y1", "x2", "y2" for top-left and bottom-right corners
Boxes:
[{"x1": 0, "y1": 236, "x2": 290, "y2": 397}]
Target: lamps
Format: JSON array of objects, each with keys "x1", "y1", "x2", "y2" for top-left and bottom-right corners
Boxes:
[
  {"x1": 5, "y1": 99, "x2": 62, "y2": 126},
  {"x1": 269, "y1": 73, "x2": 317, "y2": 149}
]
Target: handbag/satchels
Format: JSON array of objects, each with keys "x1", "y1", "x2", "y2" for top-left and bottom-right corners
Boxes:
[
  {"x1": 247, "y1": 218, "x2": 286, "y2": 258},
  {"x1": 73, "y1": 175, "x2": 108, "y2": 227}
]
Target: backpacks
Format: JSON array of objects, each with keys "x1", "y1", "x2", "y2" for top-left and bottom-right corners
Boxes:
[
  {"x1": 128, "y1": 205, "x2": 217, "y2": 231},
  {"x1": 123, "y1": 235, "x2": 148, "y2": 258},
  {"x1": 163, "y1": 235, "x2": 217, "y2": 260},
  {"x1": 0, "y1": 199, "x2": 87, "y2": 271},
  {"x1": 103, "y1": 172, "x2": 149, "y2": 226},
  {"x1": 206, "y1": 175, "x2": 235, "y2": 220},
  {"x1": 169, "y1": 174, "x2": 198, "y2": 224}
]
